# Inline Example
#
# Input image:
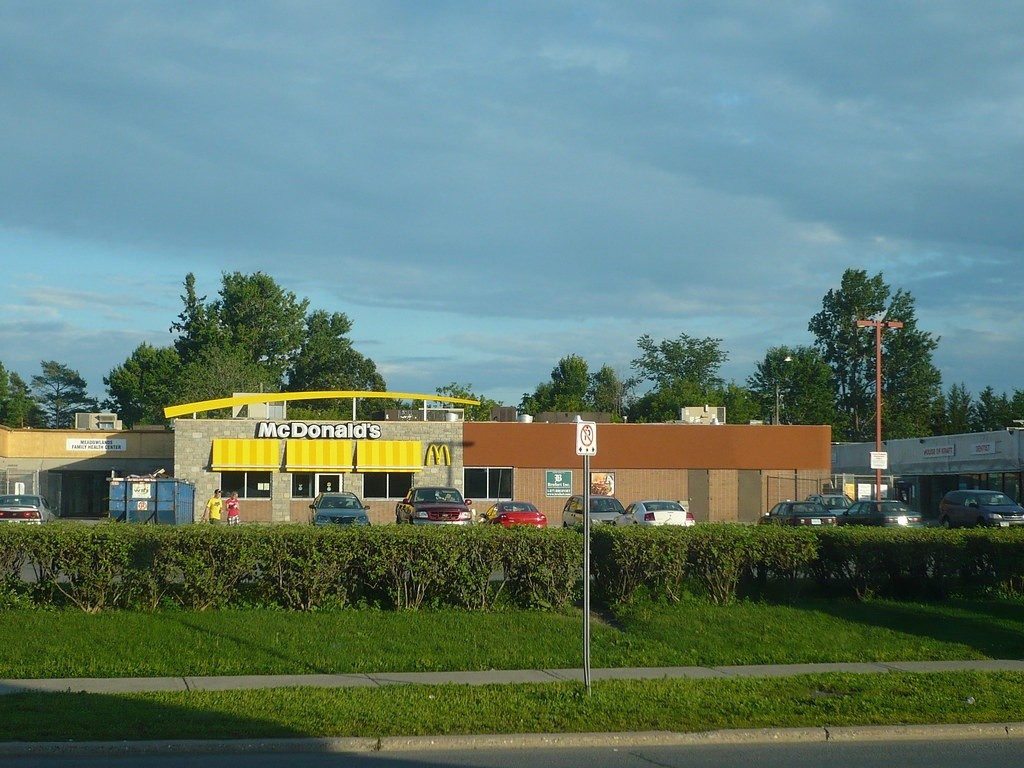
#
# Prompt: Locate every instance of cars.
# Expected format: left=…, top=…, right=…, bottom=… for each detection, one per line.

left=612, top=499, right=697, bottom=528
left=307, top=492, right=373, bottom=529
left=478, top=500, right=548, bottom=530
left=753, top=500, right=838, bottom=528
left=836, top=499, right=925, bottom=529
left=0, top=493, right=57, bottom=524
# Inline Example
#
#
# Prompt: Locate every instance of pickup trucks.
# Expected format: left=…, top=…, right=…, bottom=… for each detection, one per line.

left=393, top=486, right=475, bottom=525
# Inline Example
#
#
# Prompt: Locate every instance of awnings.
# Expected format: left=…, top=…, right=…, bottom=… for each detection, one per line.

left=356, top=440, right=424, bottom=472
left=285, top=439, right=354, bottom=472
left=211, top=439, right=281, bottom=471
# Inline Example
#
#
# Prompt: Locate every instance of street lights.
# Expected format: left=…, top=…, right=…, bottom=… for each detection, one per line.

left=855, top=320, right=904, bottom=502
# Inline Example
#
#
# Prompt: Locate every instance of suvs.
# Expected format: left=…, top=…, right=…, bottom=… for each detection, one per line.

left=802, top=494, right=868, bottom=525
left=937, top=489, right=1024, bottom=531
left=561, top=494, right=631, bottom=532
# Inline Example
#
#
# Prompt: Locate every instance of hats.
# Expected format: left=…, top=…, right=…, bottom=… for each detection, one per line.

left=215, top=489, right=222, bottom=494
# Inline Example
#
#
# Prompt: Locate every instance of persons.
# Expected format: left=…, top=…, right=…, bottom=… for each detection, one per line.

left=226, top=491, right=241, bottom=526
left=202, top=489, right=223, bottom=525
